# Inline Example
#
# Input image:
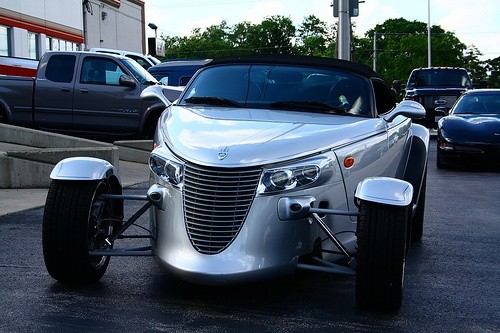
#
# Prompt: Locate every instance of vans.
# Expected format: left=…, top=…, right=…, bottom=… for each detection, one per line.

left=146, top=58, right=213, bottom=88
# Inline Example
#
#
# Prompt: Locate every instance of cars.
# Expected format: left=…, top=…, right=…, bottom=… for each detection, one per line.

left=435, top=87, right=500, bottom=170
left=41, top=53, right=428, bottom=313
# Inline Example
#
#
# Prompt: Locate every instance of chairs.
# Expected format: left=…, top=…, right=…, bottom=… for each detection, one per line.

left=52, top=57, right=67, bottom=82
left=95, top=61, right=106, bottom=83
left=269, top=69, right=304, bottom=102
left=224, top=71, right=262, bottom=100
left=302, top=74, right=338, bottom=103
left=327, top=77, right=397, bottom=117
left=467, top=101, right=487, bottom=113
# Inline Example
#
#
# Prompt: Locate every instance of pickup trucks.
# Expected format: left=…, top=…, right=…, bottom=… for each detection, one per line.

left=0, top=47, right=169, bottom=142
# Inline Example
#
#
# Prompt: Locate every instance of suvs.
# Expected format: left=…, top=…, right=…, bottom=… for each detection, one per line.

left=392, top=67, right=487, bottom=129
